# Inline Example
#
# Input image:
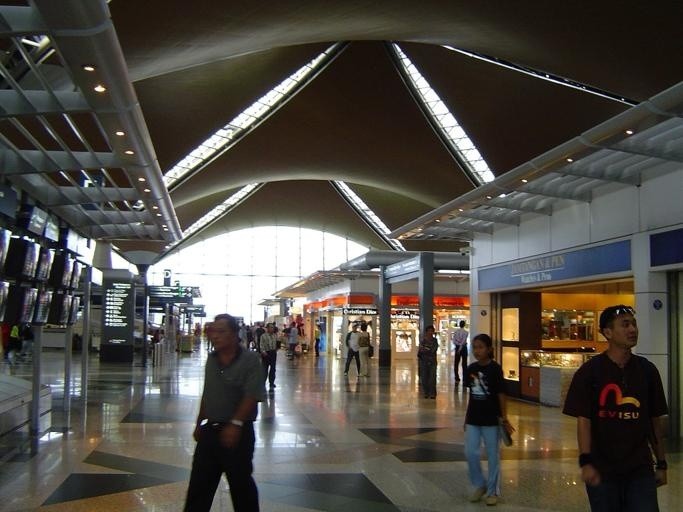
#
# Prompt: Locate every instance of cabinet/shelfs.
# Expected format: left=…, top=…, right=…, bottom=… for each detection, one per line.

left=542, top=308, right=608, bottom=343
left=490, top=295, right=542, bottom=405
left=519, top=348, right=601, bottom=407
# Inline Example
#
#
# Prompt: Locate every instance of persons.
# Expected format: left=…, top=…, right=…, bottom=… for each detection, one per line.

left=358, top=323, right=372, bottom=376
left=182, top=314, right=266, bottom=512
left=452, top=320, right=469, bottom=385
left=343, top=324, right=361, bottom=376
left=460, top=334, right=516, bottom=505
left=153, top=328, right=159, bottom=342
left=419, top=325, right=437, bottom=400
left=563, top=304, right=669, bottom=512
left=314, top=324, right=322, bottom=357
left=0, top=320, right=34, bottom=357
left=191, top=318, right=301, bottom=389
left=160, top=329, right=166, bottom=340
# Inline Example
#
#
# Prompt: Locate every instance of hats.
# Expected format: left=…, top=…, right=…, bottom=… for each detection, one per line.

left=352, top=323, right=358, bottom=328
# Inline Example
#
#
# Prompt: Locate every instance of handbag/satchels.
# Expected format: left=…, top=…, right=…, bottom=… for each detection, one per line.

left=294, top=345, right=302, bottom=355
left=499, top=416, right=513, bottom=446
left=360, top=335, right=369, bottom=347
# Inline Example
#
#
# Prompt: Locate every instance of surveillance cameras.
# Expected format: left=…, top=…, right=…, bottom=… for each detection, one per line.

left=459, top=247, right=472, bottom=257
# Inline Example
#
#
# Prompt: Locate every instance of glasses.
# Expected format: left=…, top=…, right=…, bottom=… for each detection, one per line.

left=610, top=306, right=634, bottom=318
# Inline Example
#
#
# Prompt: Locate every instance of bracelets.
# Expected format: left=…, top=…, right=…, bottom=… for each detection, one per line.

left=655, top=459, right=668, bottom=471
left=578, top=452, right=592, bottom=468
left=227, top=418, right=244, bottom=427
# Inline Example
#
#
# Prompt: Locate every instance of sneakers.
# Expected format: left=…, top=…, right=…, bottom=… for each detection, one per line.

left=425, top=394, right=436, bottom=399
left=270, top=383, right=276, bottom=387
left=344, top=372, right=370, bottom=377
left=469, top=488, right=486, bottom=502
left=485, top=494, right=498, bottom=505
